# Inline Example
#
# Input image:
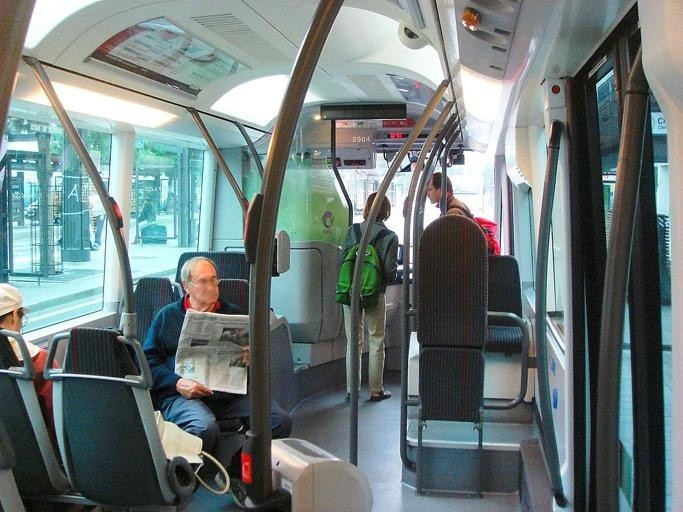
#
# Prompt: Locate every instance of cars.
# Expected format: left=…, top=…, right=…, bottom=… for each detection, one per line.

left=5, top=183, right=181, bottom=224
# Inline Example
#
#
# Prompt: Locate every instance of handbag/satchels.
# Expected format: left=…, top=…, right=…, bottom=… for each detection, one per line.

left=153, top=409, right=232, bottom=496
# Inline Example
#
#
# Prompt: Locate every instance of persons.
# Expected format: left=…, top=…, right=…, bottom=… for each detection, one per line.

left=425, top=170, right=473, bottom=223
left=138, top=257, right=291, bottom=493
left=89, top=190, right=107, bottom=251
left=130, top=197, right=157, bottom=245
left=336, top=191, right=398, bottom=402
left=0, top=284, right=63, bottom=448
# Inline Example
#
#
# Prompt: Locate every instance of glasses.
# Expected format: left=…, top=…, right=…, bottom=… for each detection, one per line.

left=187, top=279, right=219, bottom=288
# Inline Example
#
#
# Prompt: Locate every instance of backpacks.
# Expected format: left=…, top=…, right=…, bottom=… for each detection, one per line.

left=334, top=221, right=394, bottom=310
left=447, top=202, right=501, bottom=258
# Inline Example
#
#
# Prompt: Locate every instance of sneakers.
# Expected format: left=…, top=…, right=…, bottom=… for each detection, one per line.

left=346, top=392, right=359, bottom=403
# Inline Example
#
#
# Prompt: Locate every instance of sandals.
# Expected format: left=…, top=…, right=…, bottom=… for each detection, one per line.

left=370, top=390, right=391, bottom=401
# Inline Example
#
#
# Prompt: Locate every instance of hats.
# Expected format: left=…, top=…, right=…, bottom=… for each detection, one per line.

left=0, top=283, right=31, bottom=318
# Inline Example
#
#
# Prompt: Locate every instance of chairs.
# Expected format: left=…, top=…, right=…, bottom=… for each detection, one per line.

left=487, top=255, right=530, bottom=409
